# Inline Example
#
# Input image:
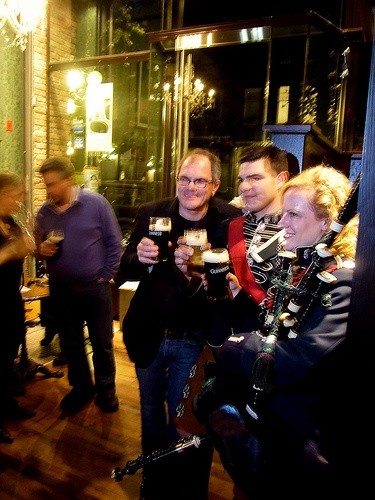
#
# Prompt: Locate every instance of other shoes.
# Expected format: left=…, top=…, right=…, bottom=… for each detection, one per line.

left=41, top=336, right=53, bottom=346
left=0, top=432, right=12, bottom=443
left=3, top=405, right=36, bottom=418
left=60, top=382, right=95, bottom=413
left=95, top=386, right=119, bottom=413
left=53, top=357, right=68, bottom=366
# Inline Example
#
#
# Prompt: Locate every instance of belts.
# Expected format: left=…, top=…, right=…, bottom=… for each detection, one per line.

left=163, top=325, right=209, bottom=343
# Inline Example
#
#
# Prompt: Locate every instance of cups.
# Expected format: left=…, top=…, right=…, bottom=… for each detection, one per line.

left=50, top=230, right=65, bottom=256
left=184, top=229, right=208, bottom=278
left=148, top=216, right=172, bottom=262
left=201, top=249, right=230, bottom=301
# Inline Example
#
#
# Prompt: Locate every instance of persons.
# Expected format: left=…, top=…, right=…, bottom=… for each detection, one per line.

left=175, top=143, right=300, bottom=379
left=117, top=147, right=245, bottom=499
left=201, top=164, right=362, bottom=500
left=33, top=157, right=123, bottom=415
left=0, top=170, right=38, bottom=445
left=39, top=299, right=67, bottom=367
left=226, top=151, right=300, bottom=213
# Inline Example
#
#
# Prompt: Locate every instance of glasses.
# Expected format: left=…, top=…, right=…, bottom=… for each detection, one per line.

left=176, top=176, right=216, bottom=188
